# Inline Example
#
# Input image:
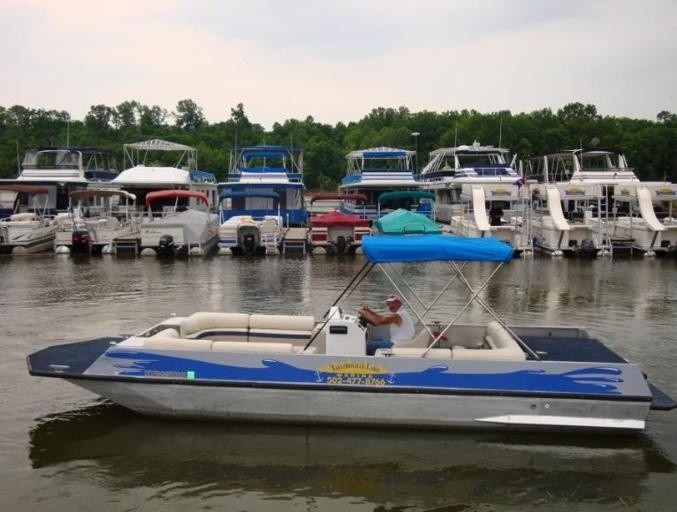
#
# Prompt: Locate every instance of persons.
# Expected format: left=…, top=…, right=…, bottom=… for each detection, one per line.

left=489, top=201, right=504, bottom=225
left=358, top=294, right=415, bottom=354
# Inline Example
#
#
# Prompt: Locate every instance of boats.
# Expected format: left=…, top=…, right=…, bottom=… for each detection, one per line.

left=24, top=207, right=676, bottom=436
left=28, top=415, right=675, bottom=503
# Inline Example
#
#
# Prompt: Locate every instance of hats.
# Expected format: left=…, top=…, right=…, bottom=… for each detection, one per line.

left=384, top=294, right=399, bottom=302
